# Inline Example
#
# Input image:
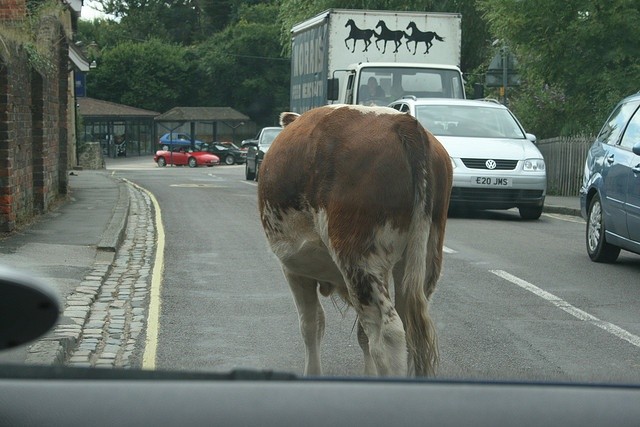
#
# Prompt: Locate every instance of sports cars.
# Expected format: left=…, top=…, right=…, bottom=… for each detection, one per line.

left=153, top=144, right=219, bottom=167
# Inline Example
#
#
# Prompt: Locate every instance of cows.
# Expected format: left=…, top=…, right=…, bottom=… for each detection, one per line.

left=255, top=102, right=454, bottom=379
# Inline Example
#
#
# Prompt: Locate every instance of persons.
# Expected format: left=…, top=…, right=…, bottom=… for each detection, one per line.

left=357, top=76, right=387, bottom=107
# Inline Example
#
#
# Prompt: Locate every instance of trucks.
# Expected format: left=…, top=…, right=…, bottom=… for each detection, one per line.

left=289, top=3, right=485, bottom=113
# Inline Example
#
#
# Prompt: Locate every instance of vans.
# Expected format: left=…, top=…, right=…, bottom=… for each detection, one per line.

left=384, top=95, right=547, bottom=218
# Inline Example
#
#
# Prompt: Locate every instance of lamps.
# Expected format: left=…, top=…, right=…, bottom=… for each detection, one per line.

left=52, top=31, right=83, bottom=48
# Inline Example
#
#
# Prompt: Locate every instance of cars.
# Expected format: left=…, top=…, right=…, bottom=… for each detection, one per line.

left=158, top=131, right=210, bottom=152
left=245, top=125, right=284, bottom=182
left=196, top=143, right=246, bottom=165
left=576, top=88, right=640, bottom=265
